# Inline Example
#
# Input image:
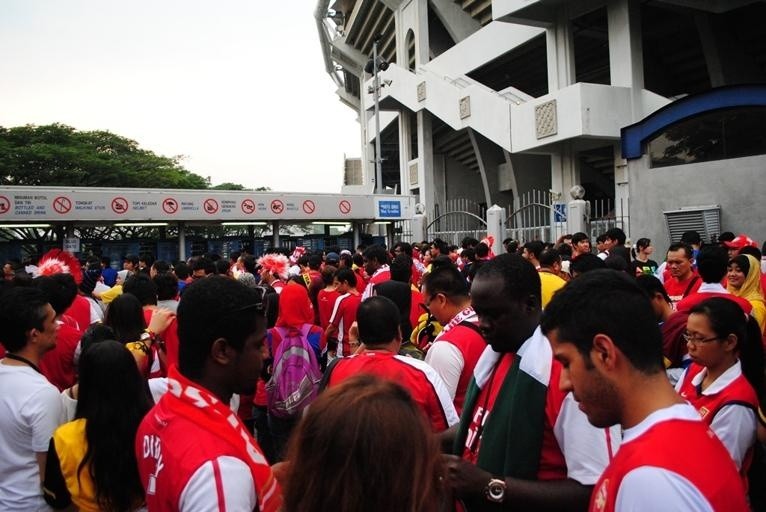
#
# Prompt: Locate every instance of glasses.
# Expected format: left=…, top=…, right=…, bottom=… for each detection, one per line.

left=240, top=285, right=269, bottom=318
left=682, top=332, right=720, bottom=345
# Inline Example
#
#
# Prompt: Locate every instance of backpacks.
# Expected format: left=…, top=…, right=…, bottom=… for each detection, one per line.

left=267, top=324, right=323, bottom=420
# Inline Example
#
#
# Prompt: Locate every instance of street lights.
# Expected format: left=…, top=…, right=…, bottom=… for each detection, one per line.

left=364, top=31, right=389, bottom=192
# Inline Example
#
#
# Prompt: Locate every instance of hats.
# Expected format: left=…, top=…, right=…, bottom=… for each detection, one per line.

left=122, top=254, right=139, bottom=263
left=238, top=272, right=258, bottom=290
left=36, top=249, right=85, bottom=285
left=257, top=254, right=291, bottom=281
left=80, top=269, right=99, bottom=295
left=309, top=255, right=323, bottom=264
left=724, top=234, right=758, bottom=248
left=326, top=253, right=343, bottom=262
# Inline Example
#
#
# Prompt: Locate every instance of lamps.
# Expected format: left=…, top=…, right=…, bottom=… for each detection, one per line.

left=568, top=185, right=586, bottom=199
left=413, top=203, right=426, bottom=215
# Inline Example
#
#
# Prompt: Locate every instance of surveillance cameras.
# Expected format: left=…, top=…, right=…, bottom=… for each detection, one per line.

left=375, top=54, right=390, bottom=71
left=363, top=60, right=373, bottom=73
